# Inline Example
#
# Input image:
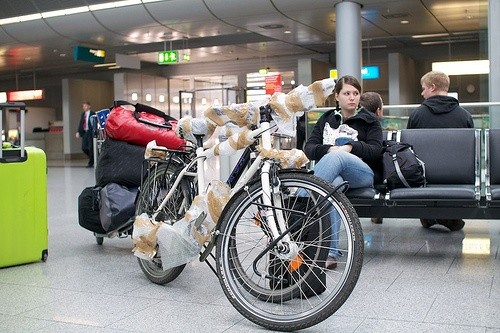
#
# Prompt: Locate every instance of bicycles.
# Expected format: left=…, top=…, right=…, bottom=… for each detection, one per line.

left=131, top=79, right=366, bottom=332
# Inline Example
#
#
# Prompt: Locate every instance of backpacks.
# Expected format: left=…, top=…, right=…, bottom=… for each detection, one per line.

left=381, top=140, right=425, bottom=191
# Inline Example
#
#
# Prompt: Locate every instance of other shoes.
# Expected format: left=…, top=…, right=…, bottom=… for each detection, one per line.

left=438, top=219, right=465, bottom=230
left=87, top=164, right=94, bottom=168
left=371, top=218, right=383, bottom=224
left=419, top=218, right=436, bottom=227
left=325, top=255, right=337, bottom=269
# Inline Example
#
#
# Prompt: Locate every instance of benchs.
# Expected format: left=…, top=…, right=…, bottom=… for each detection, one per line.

left=297, top=127, right=500, bottom=220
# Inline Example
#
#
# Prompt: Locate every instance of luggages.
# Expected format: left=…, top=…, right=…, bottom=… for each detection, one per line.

left=0, top=102, right=49, bottom=268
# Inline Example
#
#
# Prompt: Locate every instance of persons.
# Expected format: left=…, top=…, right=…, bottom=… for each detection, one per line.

left=406, top=72, right=474, bottom=232
left=288, top=75, right=386, bottom=271
left=75, top=102, right=97, bottom=168
left=360, top=91, right=384, bottom=120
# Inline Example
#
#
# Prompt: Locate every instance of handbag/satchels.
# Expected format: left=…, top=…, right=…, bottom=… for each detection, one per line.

left=99, top=182, right=141, bottom=235
left=269, top=196, right=330, bottom=298
left=78, top=187, right=105, bottom=235
left=95, top=138, right=149, bottom=187
left=105, top=101, right=186, bottom=150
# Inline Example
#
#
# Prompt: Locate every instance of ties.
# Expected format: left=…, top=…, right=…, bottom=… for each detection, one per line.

left=83, top=114, right=87, bottom=128
